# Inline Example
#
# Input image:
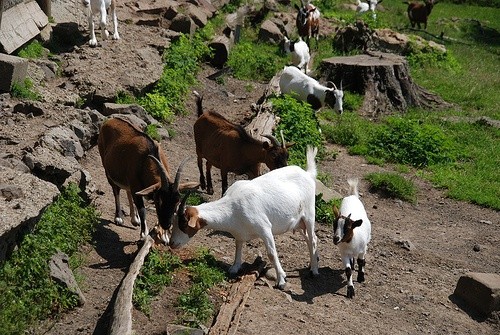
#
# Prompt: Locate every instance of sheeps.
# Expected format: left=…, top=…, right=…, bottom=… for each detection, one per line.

left=400, top=0, right=440, bottom=31
left=331, top=175, right=373, bottom=299
left=278, top=64, right=346, bottom=136
left=278, top=36, right=311, bottom=76
left=93, top=116, right=201, bottom=246
left=168, top=143, right=322, bottom=291
left=292, top=0, right=321, bottom=55
left=79, top=0, right=124, bottom=48
left=190, top=89, right=298, bottom=199
left=352, top=0, right=384, bottom=20
